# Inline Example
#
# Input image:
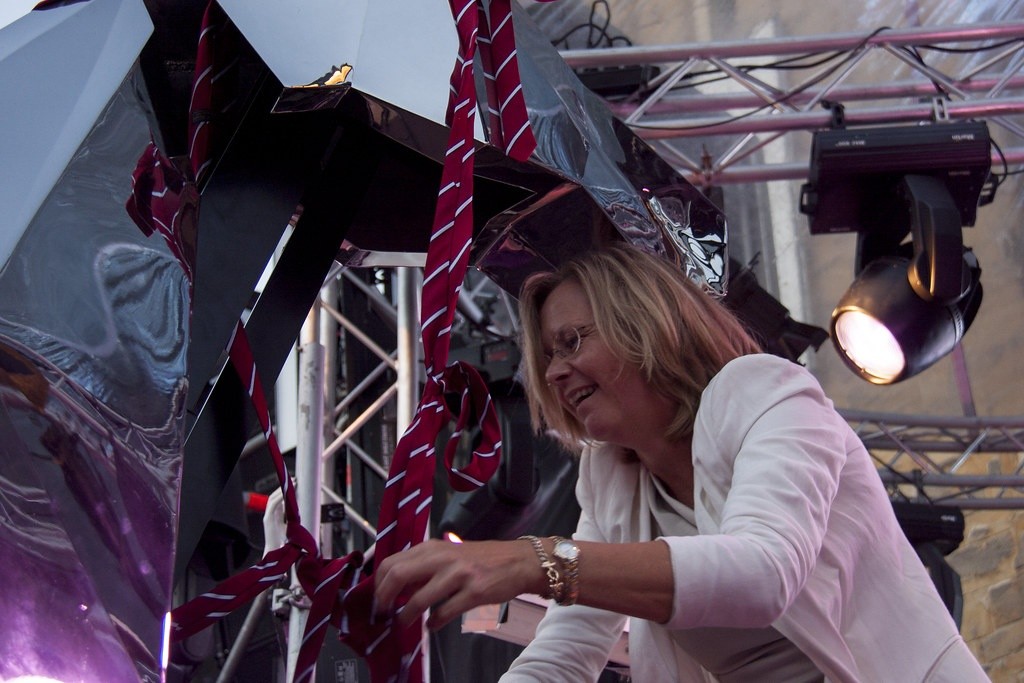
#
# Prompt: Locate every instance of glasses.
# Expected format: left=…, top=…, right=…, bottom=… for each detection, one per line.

left=544, top=322, right=599, bottom=371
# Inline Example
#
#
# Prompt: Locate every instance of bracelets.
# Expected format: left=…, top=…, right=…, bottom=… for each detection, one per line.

left=517, top=534, right=563, bottom=600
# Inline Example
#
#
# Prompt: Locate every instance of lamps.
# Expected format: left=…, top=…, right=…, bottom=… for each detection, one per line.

left=824, top=170, right=985, bottom=386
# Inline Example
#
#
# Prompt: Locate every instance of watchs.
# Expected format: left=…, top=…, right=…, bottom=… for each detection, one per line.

left=549, top=536, right=580, bottom=606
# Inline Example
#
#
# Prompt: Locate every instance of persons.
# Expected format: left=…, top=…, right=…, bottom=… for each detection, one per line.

left=373, top=242, right=993, bottom=683
